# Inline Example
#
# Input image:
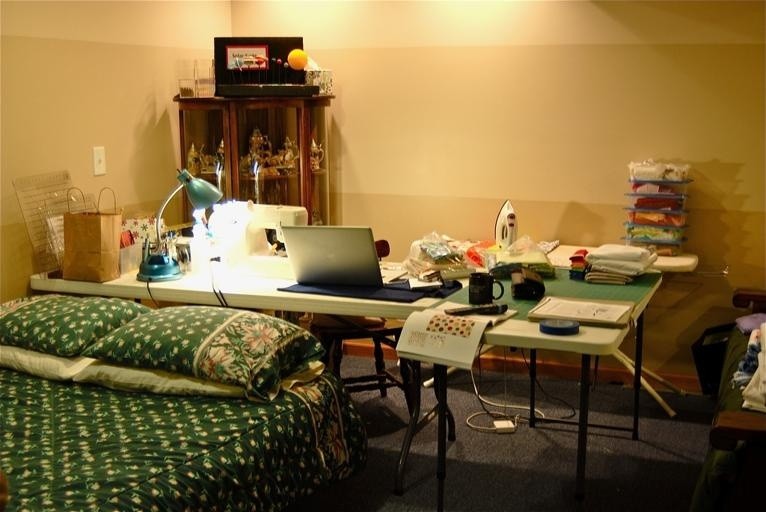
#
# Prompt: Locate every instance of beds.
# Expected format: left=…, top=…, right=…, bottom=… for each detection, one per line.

left=1, top=365, right=369, bottom=512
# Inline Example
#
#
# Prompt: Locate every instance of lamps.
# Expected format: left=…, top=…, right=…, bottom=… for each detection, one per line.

left=136, top=168, right=223, bottom=282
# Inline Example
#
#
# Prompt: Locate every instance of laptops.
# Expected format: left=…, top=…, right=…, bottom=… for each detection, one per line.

left=283, top=226, right=406, bottom=291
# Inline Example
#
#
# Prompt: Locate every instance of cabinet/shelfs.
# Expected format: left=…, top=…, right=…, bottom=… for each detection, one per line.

left=173, top=94, right=337, bottom=226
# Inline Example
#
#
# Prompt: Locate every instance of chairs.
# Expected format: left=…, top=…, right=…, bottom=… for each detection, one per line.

left=284, top=312, right=414, bottom=416
left=685, top=287, right=766, bottom=509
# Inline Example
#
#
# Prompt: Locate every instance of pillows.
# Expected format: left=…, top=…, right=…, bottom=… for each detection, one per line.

left=83, top=306, right=325, bottom=397
left=0, top=293, right=153, bottom=358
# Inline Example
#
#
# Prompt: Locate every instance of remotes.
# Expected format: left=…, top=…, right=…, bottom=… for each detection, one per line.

left=444, top=303, right=510, bottom=319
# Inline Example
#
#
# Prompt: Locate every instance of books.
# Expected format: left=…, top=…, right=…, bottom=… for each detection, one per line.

left=529, top=294, right=634, bottom=327
left=396, top=300, right=518, bottom=370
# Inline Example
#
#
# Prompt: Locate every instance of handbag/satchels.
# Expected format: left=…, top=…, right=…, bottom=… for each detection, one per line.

left=511, top=267, right=545, bottom=301
left=62, top=188, right=123, bottom=282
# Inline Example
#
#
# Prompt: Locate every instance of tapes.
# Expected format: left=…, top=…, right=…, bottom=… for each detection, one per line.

left=539, top=320, right=579, bottom=335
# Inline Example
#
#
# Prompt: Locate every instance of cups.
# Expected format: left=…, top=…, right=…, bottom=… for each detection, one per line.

left=312, top=71, right=333, bottom=94
left=179, top=80, right=194, bottom=98
left=192, top=59, right=215, bottom=98
left=469, top=273, right=504, bottom=304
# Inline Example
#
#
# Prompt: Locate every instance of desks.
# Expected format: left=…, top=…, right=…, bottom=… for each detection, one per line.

left=30, top=242, right=490, bottom=497
left=424, top=272, right=663, bottom=512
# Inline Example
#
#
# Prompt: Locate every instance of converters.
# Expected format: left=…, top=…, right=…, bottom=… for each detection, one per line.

left=494, top=420, right=515, bottom=434
